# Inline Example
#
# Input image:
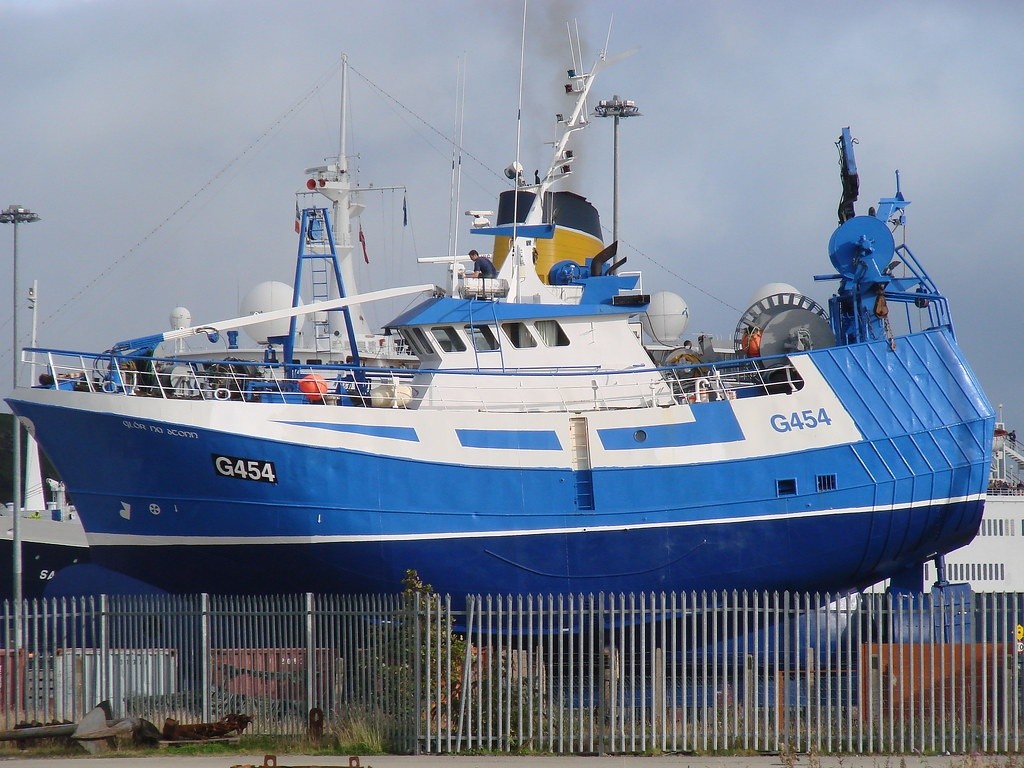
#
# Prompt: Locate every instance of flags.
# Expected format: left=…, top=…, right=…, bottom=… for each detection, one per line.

left=295, top=200, right=300, bottom=234
left=403, top=195, right=407, bottom=227
left=359, top=225, right=369, bottom=264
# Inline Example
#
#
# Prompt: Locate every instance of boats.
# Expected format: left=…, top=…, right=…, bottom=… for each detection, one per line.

left=4, top=0, right=996, bottom=640
left=687, top=402, right=1024, bottom=665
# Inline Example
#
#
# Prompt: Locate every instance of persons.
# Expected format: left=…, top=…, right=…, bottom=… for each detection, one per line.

left=465, top=250, right=496, bottom=301
left=1009, top=430, right=1016, bottom=450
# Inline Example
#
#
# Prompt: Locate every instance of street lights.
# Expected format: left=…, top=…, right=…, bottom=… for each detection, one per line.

left=0, top=202, right=40, bottom=649
